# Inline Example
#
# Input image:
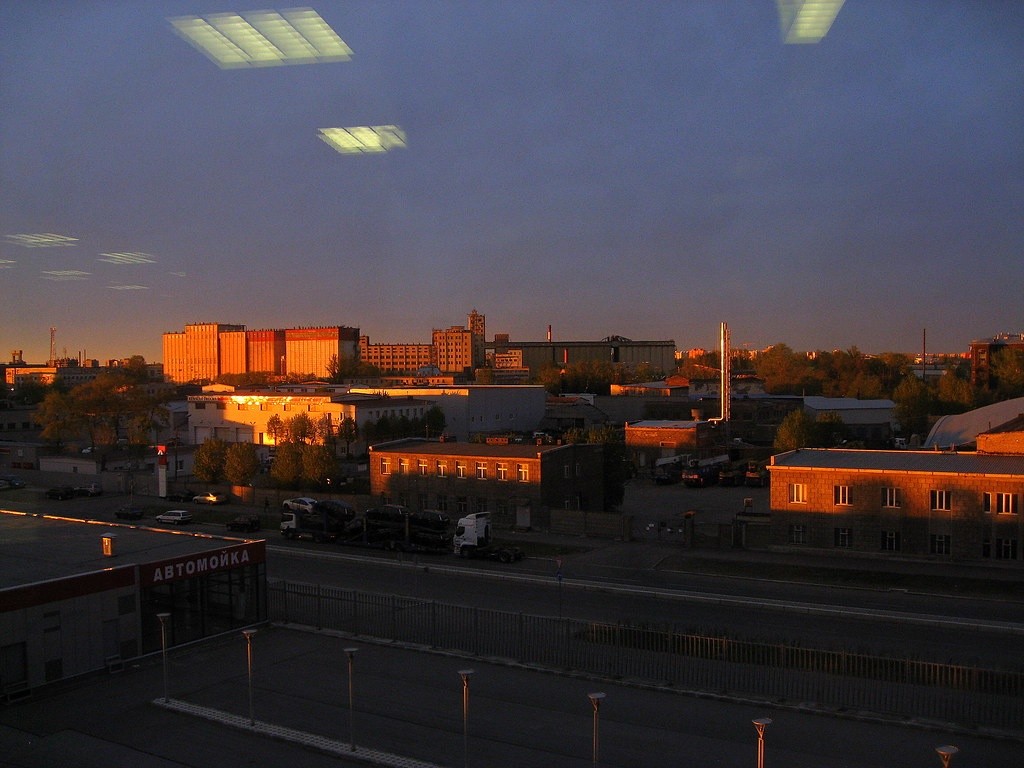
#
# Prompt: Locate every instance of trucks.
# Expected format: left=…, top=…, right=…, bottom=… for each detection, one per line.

left=656, top=449, right=770, bottom=488
left=450, top=512, right=528, bottom=564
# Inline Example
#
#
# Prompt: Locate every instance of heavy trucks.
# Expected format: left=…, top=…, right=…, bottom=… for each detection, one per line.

left=278, top=496, right=456, bottom=556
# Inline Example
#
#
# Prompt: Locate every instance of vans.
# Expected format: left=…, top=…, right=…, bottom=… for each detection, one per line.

left=1, top=474, right=26, bottom=489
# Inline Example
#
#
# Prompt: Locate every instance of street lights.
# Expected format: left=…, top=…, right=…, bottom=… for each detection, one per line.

left=587, top=692, right=608, bottom=768
left=750, top=717, right=773, bottom=768
left=456, top=669, right=480, bottom=768
left=156, top=610, right=171, bottom=705
left=241, top=628, right=258, bottom=725
left=343, top=646, right=359, bottom=754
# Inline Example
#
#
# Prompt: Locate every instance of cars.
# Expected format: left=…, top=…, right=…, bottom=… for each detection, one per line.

left=114, top=506, right=145, bottom=519
left=156, top=510, right=193, bottom=525
left=44, top=481, right=101, bottom=501
left=226, top=515, right=261, bottom=533
left=364, top=504, right=411, bottom=524
left=313, top=499, right=357, bottom=522
left=281, top=498, right=316, bottom=514
left=408, top=509, right=451, bottom=531
left=192, top=492, right=228, bottom=505
